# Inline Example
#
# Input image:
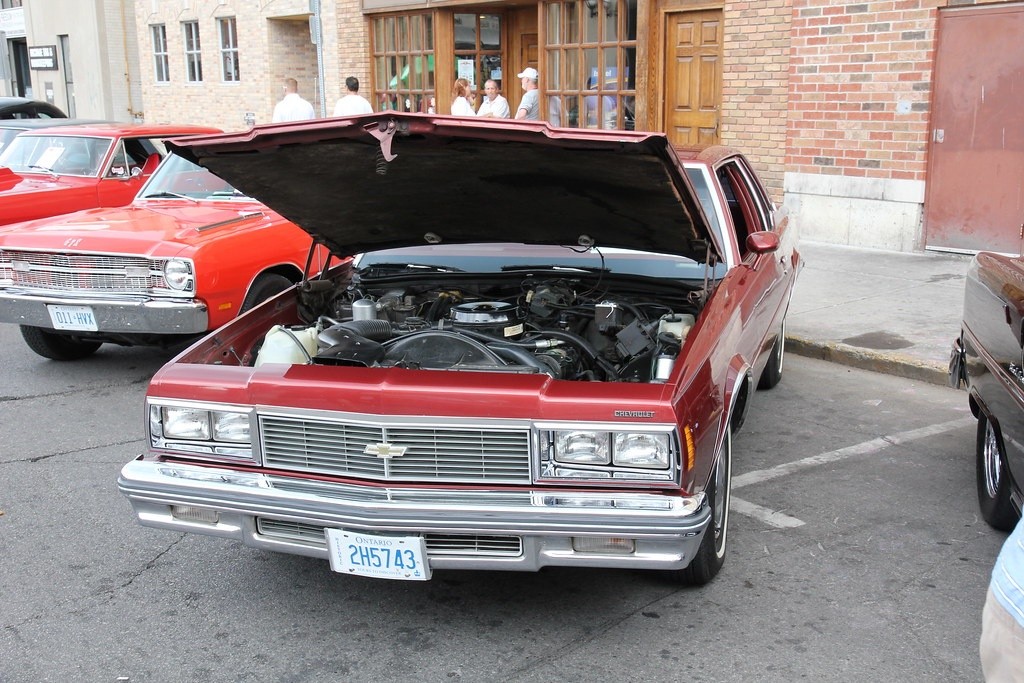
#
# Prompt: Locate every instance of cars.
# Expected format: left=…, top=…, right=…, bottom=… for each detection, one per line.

left=948, top=255, right=1024, bottom=531
left=0, top=96, right=226, bottom=234
left=115, top=109, right=805, bottom=589
left=0, top=144, right=352, bottom=364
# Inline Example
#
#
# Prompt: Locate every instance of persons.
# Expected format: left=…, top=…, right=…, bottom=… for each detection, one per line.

left=476, top=79, right=511, bottom=119
left=578, top=77, right=614, bottom=131
left=271, top=78, right=316, bottom=124
left=515, top=68, right=541, bottom=120
left=333, top=77, right=374, bottom=118
left=548, top=94, right=569, bottom=128
left=969, top=505, right=1024, bottom=683
left=450, top=78, right=478, bottom=117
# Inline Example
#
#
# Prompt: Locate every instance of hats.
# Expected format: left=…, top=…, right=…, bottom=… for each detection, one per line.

left=517, top=68, right=538, bottom=79
